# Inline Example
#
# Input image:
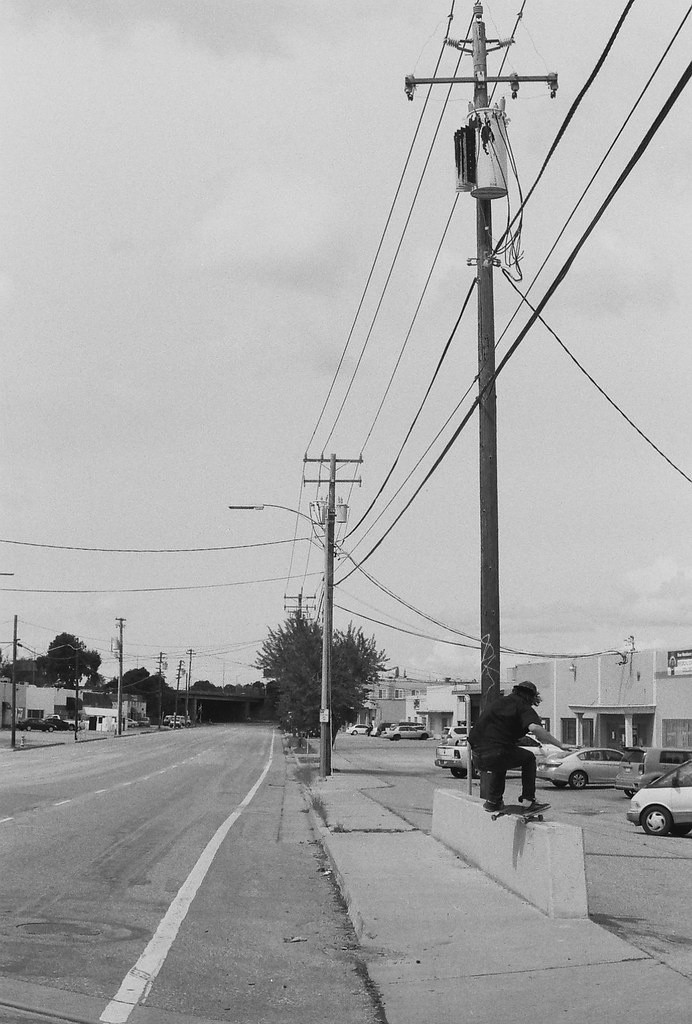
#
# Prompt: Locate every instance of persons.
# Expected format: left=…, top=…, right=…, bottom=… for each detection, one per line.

left=366, top=723, right=373, bottom=736
left=468, top=681, right=583, bottom=811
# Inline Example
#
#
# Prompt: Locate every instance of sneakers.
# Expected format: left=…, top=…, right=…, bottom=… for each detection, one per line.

left=523, top=803, right=551, bottom=816
left=483, top=799, right=505, bottom=810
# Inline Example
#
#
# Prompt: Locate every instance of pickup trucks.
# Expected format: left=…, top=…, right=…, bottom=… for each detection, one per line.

left=435, top=737, right=489, bottom=779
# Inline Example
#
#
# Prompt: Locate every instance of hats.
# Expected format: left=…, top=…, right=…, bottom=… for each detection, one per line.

left=514, top=682, right=536, bottom=697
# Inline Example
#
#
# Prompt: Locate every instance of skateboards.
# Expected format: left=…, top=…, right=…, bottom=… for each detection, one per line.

left=485, top=803, right=551, bottom=824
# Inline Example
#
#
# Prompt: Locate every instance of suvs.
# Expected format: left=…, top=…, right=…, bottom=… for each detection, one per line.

left=164, top=715, right=191, bottom=728
left=441, top=726, right=473, bottom=744
left=139, top=717, right=151, bottom=727
left=170, top=716, right=181, bottom=728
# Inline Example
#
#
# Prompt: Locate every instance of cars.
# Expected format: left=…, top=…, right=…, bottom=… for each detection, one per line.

left=16, top=718, right=57, bottom=732
left=128, top=718, right=140, bottom=727
left=39, top=718, right=75, bottom=732
left=346, top=724, right=370, bottom=735
left=380, top=726, right=430, bottom=741
left=536, top=747, right=627, bottom=789
left=507, top=734, right=548, bottom=771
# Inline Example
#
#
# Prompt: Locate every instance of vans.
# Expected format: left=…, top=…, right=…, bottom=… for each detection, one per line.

left=615, top=747, right=692, bottom=798
left=627, top=759, right=692, bottom=836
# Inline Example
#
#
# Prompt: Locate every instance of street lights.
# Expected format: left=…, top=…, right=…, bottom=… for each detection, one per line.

left=227, top=504, right=328, bottom=779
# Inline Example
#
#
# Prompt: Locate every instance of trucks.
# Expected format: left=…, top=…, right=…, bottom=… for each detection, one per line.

left=42, top=715, right=79, bottom=731
